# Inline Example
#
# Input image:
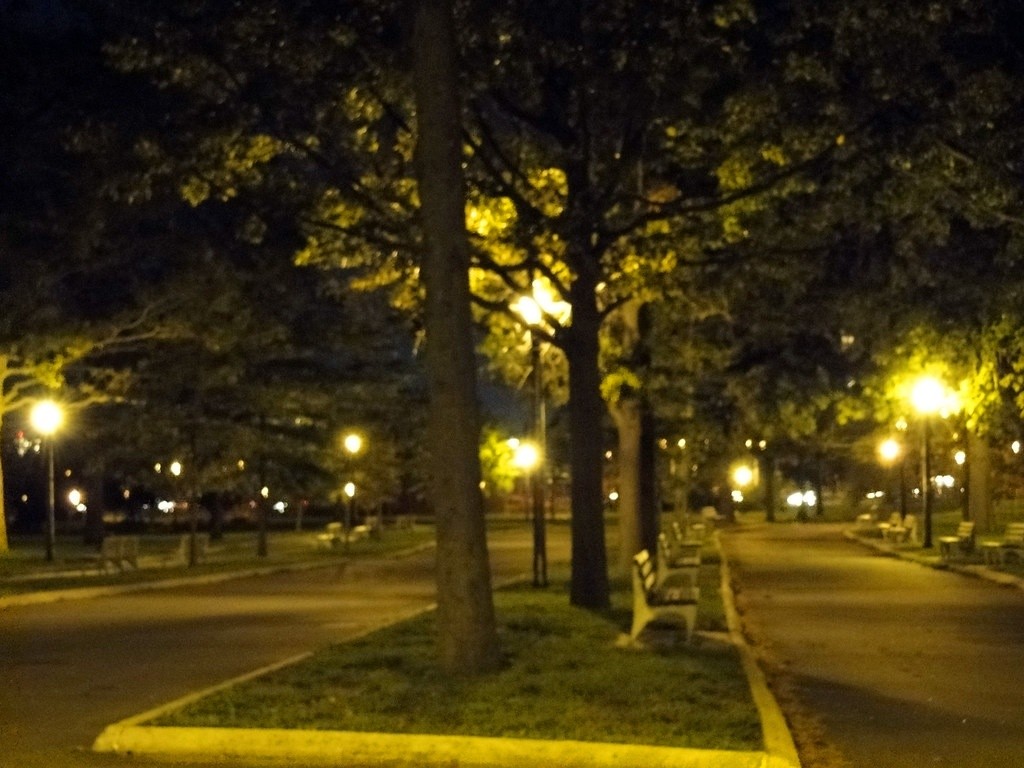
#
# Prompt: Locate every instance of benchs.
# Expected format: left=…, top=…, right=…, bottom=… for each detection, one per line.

left=74, top=536, right=140, bottom=578
left=317, top=512, right=418, bottom=548
left=671, top=522, right=703, bottom=557
left=653, top=533, right=699, bottom=592
left=856, top=505, right=878, bottom=527
left=980, top=523, right=1024, bottom=566
left=890, top=513, right=918, bottom=544
left=686, top=506, right=721, bottom=536
left=938, top=521, right=975, bottom=561
left=631, top=549, right=700, bottom=639
left=170, top=534, right=210, bottom=565
left=878, top=512, right=902, bottom=540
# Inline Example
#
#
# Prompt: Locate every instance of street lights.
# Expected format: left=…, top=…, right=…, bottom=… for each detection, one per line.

left=334, top=426, right=371, bottom=531
left=912, top=374, right=950, bottom=548
left=512, top=284, right=569, bottom=592
left=25, top=394, right=70, bottom=566
